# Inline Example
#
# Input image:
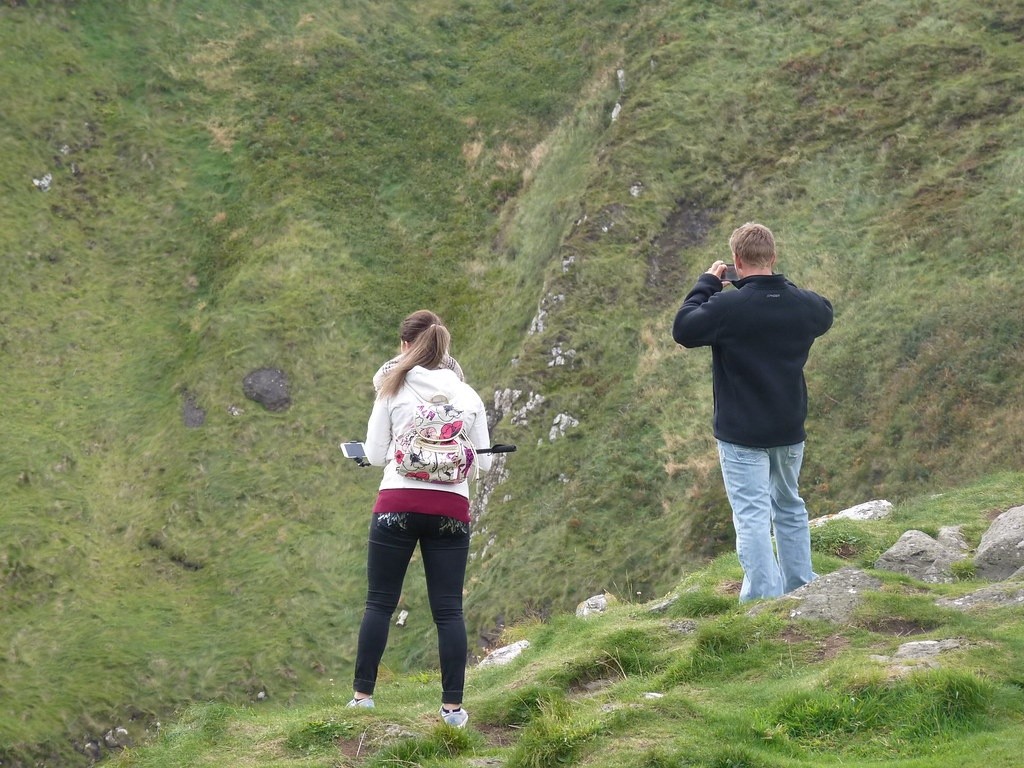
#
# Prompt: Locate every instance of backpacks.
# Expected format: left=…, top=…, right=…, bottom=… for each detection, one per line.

left=391, top=378, right=480, bottom=485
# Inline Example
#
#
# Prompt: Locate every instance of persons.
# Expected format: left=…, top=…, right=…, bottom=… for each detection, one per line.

left=345, top=310, right=492, bottom=731
left=672, top=223, right=834, bottom=606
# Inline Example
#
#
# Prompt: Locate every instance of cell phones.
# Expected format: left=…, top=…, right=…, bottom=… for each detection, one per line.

left=340, top=442, right=366, bottom=459
left=720, top=264, right=738, bottom=280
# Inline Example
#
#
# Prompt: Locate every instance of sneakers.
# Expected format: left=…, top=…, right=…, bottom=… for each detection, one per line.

left=346, top=697, right=374, bottom=708
left=438, top=704, right=468, bottom=730
